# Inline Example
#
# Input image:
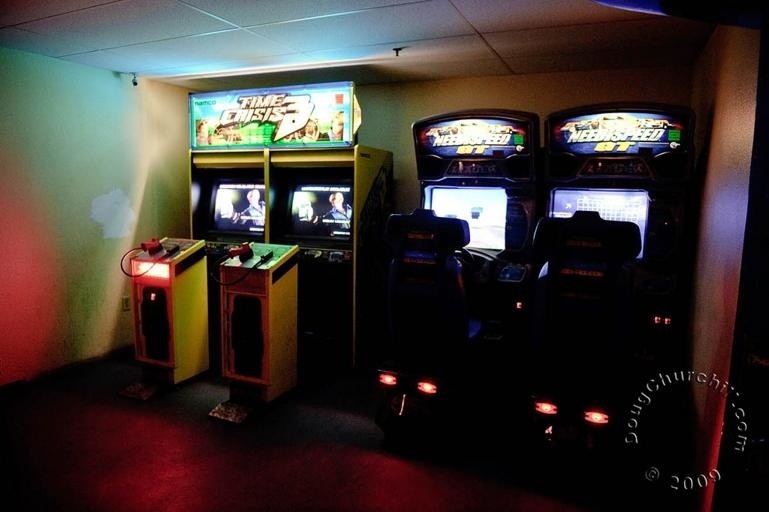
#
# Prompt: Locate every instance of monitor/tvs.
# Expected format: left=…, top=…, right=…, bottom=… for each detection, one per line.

left=209, top=181, right=266, bottom=233
left=287, top=184, right=353, bottom=244
left=550, top=189, right=648, bottom=258
left=430, top=187, right=508, bottom=250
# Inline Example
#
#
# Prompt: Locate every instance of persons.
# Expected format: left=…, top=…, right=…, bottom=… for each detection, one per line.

left=298, top=191, right=353, bottom=226
left=221, top=188, right=266, bottom=228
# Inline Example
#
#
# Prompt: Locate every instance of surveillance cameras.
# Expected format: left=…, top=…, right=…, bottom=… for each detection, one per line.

left=132, top=74, right=138, bottom=87
